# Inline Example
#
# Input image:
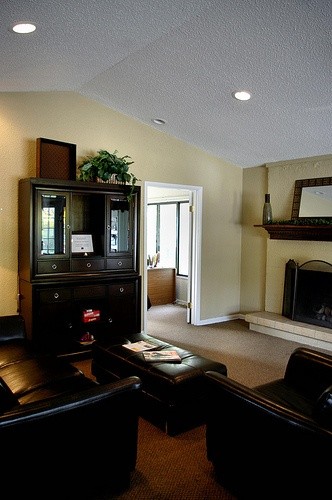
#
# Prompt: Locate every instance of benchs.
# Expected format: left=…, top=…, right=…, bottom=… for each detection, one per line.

left=90, top=333, right=227, bottom=436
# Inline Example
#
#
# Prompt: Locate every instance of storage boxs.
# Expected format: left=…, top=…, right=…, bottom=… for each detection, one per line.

left=34, top=138, right=77, bottom=180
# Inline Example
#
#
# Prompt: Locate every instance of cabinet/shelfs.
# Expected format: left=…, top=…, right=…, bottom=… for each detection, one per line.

left=18, top=177, right=141, bottom=363
left=147, top=267, right=176, bottom=305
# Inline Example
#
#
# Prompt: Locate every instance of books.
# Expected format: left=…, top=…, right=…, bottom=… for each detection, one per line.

left=142, top=350, right=181, bottom=362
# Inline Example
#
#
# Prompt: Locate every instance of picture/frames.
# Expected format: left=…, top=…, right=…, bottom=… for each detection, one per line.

left=291, top=177, right=332, bottom=219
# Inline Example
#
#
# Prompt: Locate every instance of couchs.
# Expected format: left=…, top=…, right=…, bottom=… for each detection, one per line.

left=203, top=346, right=332, bottom=500
left=0, top=314, right=142, bottom=500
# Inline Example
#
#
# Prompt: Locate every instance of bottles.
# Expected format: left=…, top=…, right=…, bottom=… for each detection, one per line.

left=263, top=193, right=273, bottom=224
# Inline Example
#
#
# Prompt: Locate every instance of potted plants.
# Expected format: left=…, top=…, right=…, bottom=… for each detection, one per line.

left=77, top=149, right=141, bottom=203
